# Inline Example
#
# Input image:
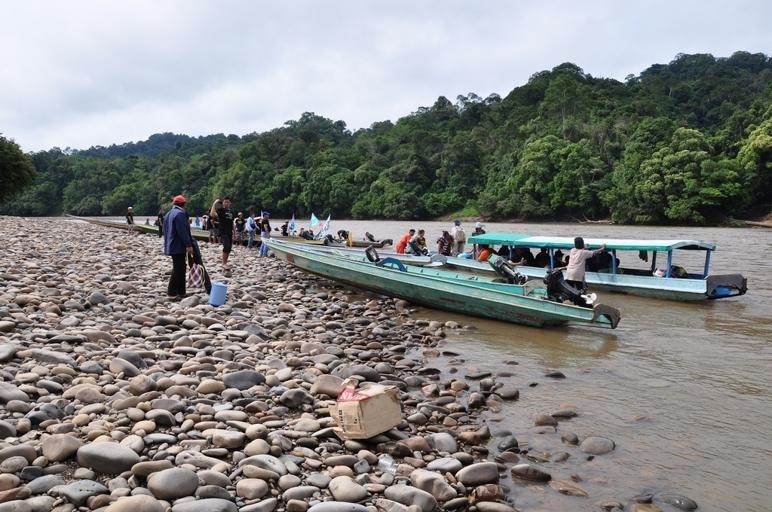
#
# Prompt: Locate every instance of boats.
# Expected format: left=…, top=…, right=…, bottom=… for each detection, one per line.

left=259, top=237, right=624, bottom=329
left=436, top=229, right=747, bottom=302
left=65, top=213, right=449, bottom=297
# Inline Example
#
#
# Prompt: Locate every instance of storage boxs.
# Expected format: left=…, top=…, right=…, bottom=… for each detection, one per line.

left=329, top=380, right=403, bottom=441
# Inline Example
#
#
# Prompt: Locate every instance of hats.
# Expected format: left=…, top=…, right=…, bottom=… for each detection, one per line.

left=173, top=195, right=186, bottom=203
left=263, top=211, right=270, bottom=215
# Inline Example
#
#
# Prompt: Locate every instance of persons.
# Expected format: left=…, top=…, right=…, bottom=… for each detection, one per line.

left=396, top=220, right=607, bottom=309
left=125, top=197, right=349, bottom=302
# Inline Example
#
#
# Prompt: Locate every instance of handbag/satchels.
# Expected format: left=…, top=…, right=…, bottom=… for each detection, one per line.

left=186, top=250, right=204, bottom=288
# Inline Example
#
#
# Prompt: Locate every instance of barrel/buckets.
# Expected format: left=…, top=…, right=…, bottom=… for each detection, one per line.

left=208, top=282, right=228, bottom=305
